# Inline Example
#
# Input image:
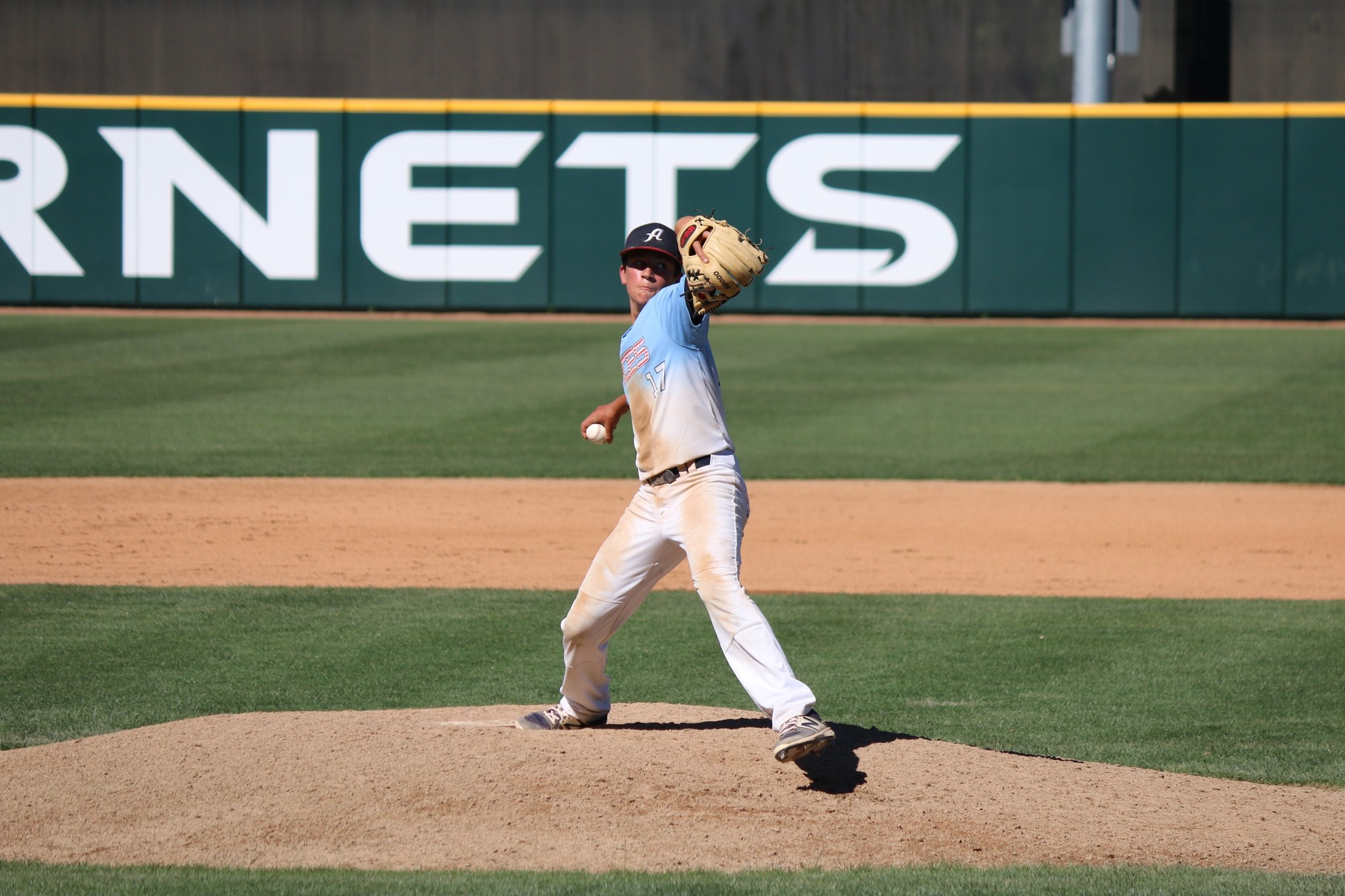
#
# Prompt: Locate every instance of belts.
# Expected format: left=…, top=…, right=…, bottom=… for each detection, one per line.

left=647, top=455, right=710, bottom=484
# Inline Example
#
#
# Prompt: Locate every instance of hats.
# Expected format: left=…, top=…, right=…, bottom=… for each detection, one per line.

left=620, top=223, right=682, bottom=264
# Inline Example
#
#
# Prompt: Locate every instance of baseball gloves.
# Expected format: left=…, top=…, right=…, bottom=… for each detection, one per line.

left=675, top=206, right=775, bottom=319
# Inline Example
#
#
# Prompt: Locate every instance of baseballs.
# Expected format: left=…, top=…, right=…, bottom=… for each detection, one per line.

left=586, top=423, right=607, bottom=445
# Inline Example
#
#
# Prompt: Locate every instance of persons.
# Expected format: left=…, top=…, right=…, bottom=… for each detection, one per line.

left=511, top=215, right=835, bottom=763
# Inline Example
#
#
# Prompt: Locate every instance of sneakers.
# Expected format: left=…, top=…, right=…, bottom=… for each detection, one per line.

left=773, top=709, right=835, bottom=763
left=514, top=704, right=607, bottom=730
